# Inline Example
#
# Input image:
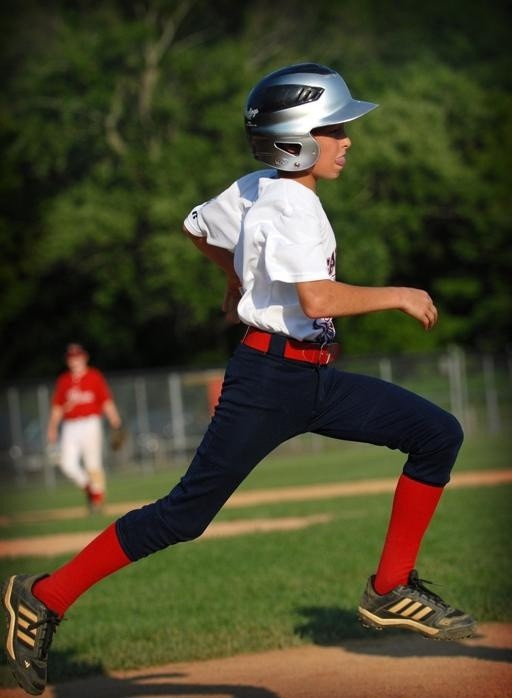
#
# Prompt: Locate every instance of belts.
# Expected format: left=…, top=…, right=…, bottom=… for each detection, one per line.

left=242, top=326, right=342, bottom=366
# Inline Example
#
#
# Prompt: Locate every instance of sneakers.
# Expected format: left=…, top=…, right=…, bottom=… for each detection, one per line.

left=356, top=569, right=479, bottom=641
left=1, top=572, right=65, bottom=696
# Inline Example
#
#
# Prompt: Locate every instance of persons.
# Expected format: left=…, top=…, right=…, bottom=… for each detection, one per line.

left=45, top=339, right=122, bottom=513
left=0, top=60, right=482, bottom=695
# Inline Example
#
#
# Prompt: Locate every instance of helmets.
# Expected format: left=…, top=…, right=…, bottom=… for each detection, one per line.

left=244, top=63, right=379, bottom=172
